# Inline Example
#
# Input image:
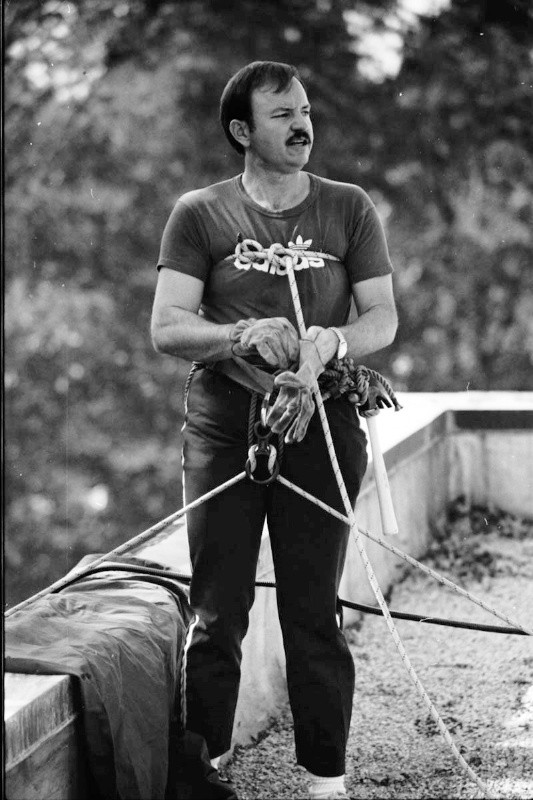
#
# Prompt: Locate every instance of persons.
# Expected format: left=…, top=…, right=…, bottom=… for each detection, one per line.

left=150, top=61, right=398, bottom=800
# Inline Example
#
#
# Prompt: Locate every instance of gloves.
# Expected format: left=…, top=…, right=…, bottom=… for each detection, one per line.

left=230, top=318, right=300, bottom=371
left=267, top=338, right=326, bottom=445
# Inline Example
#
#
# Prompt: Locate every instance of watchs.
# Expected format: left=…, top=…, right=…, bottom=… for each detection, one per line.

left=331, top=327, right=348, bottom=360
